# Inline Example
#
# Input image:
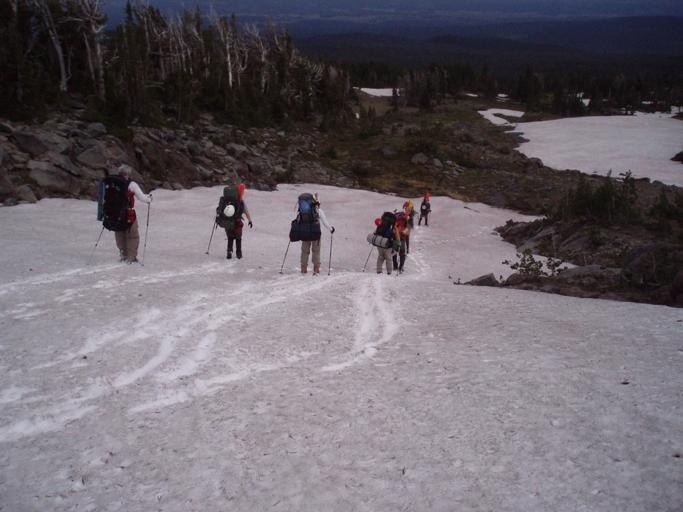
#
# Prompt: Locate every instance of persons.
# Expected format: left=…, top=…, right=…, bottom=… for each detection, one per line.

left=116, top=164, right=153, bottom=264
left=299, top=193, right=335, bottom=274
left=375, top=192, right=433, bottom=275
left=225, top=181, right=253, bottom=259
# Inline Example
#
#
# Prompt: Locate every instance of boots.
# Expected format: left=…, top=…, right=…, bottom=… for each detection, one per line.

left=391, top=252, right=398, bottom=271
left=418, top=218, right=428, bottom=226
left=398, top=255, right=405, bottom=273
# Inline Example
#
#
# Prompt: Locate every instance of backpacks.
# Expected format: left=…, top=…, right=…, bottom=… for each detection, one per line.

left=95, top=174, right=134, bottom=233
left=213, top=185, right=244, bottom=231
left=372, top=211, right=397, bottom=239
left=287, top=193, right=321, bottom=242
